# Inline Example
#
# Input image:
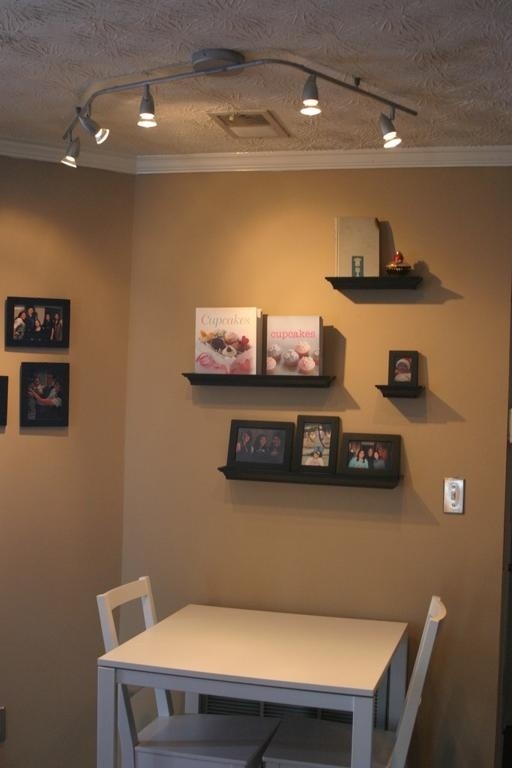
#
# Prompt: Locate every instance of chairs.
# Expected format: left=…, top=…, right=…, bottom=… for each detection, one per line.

left=96, top=575, right=283, bottom=768
left=262, top=595, right=448, bottom=768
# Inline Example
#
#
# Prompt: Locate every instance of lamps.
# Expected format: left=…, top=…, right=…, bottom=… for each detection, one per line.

left=60, top=48, right=418, bottom=168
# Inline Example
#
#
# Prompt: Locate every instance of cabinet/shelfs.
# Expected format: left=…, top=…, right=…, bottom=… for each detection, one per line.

left=181, top=276, right=427, bottom=489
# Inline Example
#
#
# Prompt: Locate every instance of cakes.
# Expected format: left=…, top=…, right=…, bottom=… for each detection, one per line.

left=266, top=342, right=320, bottom=375
left=197, top=328, right=256, bottom=376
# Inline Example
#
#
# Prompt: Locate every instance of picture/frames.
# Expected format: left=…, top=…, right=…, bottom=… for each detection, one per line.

left=0, top=375, right=8, bottom=426
left=5, top=294, right=70, bottom=349
left=19, top=361, right=69, bottom=427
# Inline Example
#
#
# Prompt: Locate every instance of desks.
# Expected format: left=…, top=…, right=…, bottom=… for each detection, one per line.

left=96, top=603, right=410, bottom=768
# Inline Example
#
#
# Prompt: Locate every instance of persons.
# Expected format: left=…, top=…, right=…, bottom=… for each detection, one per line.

left=32, top=378, right=67, bottom=407
left=12, top=304, right=63, bottom=343
left=394, top=356, right=412, bottom=383
left=235, top=429, right=393, bottom=473
left=31, top=373, right=46, bottom=397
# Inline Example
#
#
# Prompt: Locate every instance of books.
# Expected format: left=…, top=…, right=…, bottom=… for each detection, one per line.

left=332, top=213, right=382, bottom=276
left=266, top=314, right=326, bottom=376
left=194, top=305, right=264, bottom=374
